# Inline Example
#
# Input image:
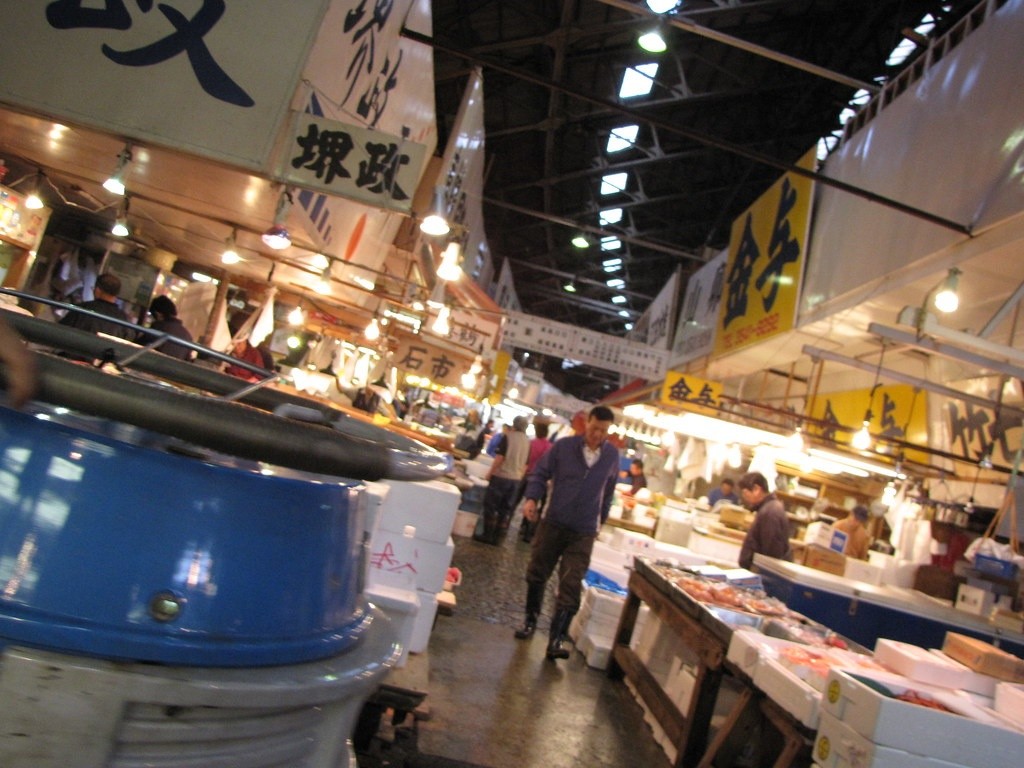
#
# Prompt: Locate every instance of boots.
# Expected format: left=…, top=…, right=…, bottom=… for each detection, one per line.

left=546, top=609, right=577, bottom=658
left=515, top=591, right=542, bottom=640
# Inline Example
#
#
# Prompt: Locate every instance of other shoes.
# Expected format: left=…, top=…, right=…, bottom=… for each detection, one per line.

left=473, top=533, right=505, bottom=547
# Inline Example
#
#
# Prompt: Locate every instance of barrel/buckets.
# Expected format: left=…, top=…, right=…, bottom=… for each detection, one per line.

left=454, top=510, right=478, bottom=539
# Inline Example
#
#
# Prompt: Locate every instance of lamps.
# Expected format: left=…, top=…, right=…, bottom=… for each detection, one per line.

left=103, top=144, right=137, bottom=195
left=934, top=266, right=962, bottom=314
left=426, top=279, right=448, bottom=308
left=638, top=25, right=667, bottom=52
left=421, top=188, right=449, bottom=236
left=264, top=188, right=291, bottom=249
left=572, top=232, right=592, bottom=249
left=111, top=198, right=131, bottom=237
left=435, top=229, right=464, bottom=282
left=564, top=279, right=575, bottom=292
left=220, top=236, right=239, bottom=264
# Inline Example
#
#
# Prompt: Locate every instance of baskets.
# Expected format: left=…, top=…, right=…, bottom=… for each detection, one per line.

left=974, top=554, right=1019, bottom=579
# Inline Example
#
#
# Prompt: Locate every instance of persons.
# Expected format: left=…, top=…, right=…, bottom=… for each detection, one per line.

left=737, top=472, right=794, bottom=575
left=56, top=273, right=137, bottom=367
left=393, top=387, right=512, bottom=459
left=135, top=294, right=194, bottom=363
left=831, top=505, right=871, bottom=562
left=709, top=479, right=740, bottom=508
left=513, top=405, right=620, bottom=662
left=473, top=415, right=531, bottom=549
left=224, top=337, right=265, bottom=383
left=618, top=459, right=646, bottom=496
left=506, top=414, right=555, bottom=543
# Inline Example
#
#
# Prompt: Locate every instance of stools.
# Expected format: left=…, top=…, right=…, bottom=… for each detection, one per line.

left=699, top=689, right=814, bottom=768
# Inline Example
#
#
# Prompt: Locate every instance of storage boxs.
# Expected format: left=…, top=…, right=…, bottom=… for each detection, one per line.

left=569, top=507, right=692, bottom=669
left=363, top=480, right=460, bottom=668
left=803, top=543, right=845, bottom=576
left=728, top=583, right=1024, bottom=768
left=806, top=523, right=849, bottom=555
left=720, top=507, right=743, bottom=527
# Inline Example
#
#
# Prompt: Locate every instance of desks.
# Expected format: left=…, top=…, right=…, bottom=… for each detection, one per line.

left=607, top=567, right=749, bottom=768
left=353, top=649, right=429, bottom=750
left=756, top=552, right=1024, bottom=656
left=431, top=590, right=455, bottom=630
